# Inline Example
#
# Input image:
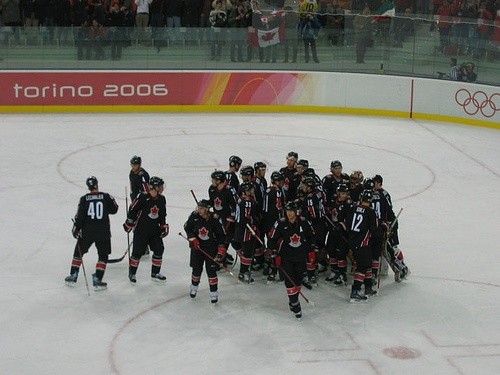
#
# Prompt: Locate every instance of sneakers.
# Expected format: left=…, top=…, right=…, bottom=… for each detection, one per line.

left=65, top=270, right=78, bottom=286
left=210, top=290, right=217, bottom=305
left=151, top=273, right=166, bottom=281
left=129, top=274, right=136, bottom=285
left=216, top=253, right=411, bottom=321
left=190, top=285, right=197, bottom=301
left=93, top=273, right=107, bottom=290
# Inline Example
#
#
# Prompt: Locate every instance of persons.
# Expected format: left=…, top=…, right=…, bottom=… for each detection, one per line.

left=208, top=151, right=411, bottom=321
left=123, top=176, right=169, bottom=286
left=183, top=199, right=228, bottom=306
left=128, top=156, right=150, bottom=259
left=64, top=176, right=118, bottom=291
left=18, top=0, right=500, bottom=84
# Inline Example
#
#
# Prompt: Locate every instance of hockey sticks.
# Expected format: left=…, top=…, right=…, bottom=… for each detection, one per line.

left=248, top=236, right=256, bottom=285
left=191, top=189, right=198, bottom=205
left=217, top=249, right=238, bottom=274
left=364, top=232, right=386, bottom=299
left=126, top=186, right=130, bottom=260
left=324, top=214, right=349, bottom=243
left=77, top=235, right=90, bottom=296
left=107, top=240, right=133, bottom=263
left=246, top=222, right=316, bottom=306
left=387, top=208, right=404, bottom=236
left=179, top=232, right=233, bottom=276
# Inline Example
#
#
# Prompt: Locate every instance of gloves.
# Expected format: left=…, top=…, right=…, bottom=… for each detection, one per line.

left=214, top=245, right=227, bottom=264
left=308, top=252, right=316, bottom=263
left=123, top=219, right=136, bottom=233
left=159, top=224, right=169, bottom=238
left=189, top=237, right=200, bottom=252
left=275, top=256, right=281, bottom=268
left=72, top=223, right=80, bottom=239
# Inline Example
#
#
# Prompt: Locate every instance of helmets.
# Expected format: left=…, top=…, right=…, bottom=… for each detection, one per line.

left=211, top=152, right=383, bottom=210
left=150, top=176, right=164, bottom=185
left=198, top=199, right=211, bottom=208
left=86, top=176, right=97, bottom=186
left=130, top=155, right=141, bottom=164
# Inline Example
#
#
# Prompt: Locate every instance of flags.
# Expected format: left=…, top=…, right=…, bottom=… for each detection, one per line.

left=370, top=1, right=395, bottom=25
left=247, top=23, right=286, bottom=48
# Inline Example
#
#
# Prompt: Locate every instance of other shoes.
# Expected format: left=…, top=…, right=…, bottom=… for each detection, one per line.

left=145, top=247, right=149, bottom=254
